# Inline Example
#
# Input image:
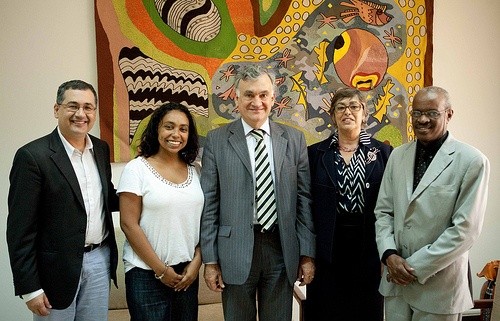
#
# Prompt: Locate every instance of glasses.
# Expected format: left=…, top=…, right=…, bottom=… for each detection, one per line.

left=408, top=108, right=447, bottom=119
left=334, top=104, right=362, bottom=114
left=58, top=103, right=97, bottom=113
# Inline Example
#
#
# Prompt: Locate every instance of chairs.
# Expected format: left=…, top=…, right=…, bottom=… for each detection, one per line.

left=462, top=261, right=500, bottom=321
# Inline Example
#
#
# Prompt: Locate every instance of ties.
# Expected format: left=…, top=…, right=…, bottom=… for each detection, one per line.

left=251, top=129, right=278, bottom=229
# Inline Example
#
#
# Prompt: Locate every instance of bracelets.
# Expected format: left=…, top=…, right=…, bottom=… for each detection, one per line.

left=155, top=264, right=169, bottom=280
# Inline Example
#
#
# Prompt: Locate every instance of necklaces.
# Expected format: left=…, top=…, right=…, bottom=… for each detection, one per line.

left=338, top=143, right=363, bottom=153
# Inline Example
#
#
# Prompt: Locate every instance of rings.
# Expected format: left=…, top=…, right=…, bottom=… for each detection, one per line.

left=312, top=276, right=314, bottom=278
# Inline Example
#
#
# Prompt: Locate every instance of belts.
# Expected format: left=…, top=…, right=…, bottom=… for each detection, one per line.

left=84, top=238, right=109, bottom=253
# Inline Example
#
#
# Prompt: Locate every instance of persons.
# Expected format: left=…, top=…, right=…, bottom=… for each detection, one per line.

left=116, top=102, right=205, bottom=321
left=306, top=86, right=395, bottom=321
left=201, top=62, right=316, bottom=321
left=6, top=79, right=120, bottom=321
left=374, top=86, right=490, bottom=321
